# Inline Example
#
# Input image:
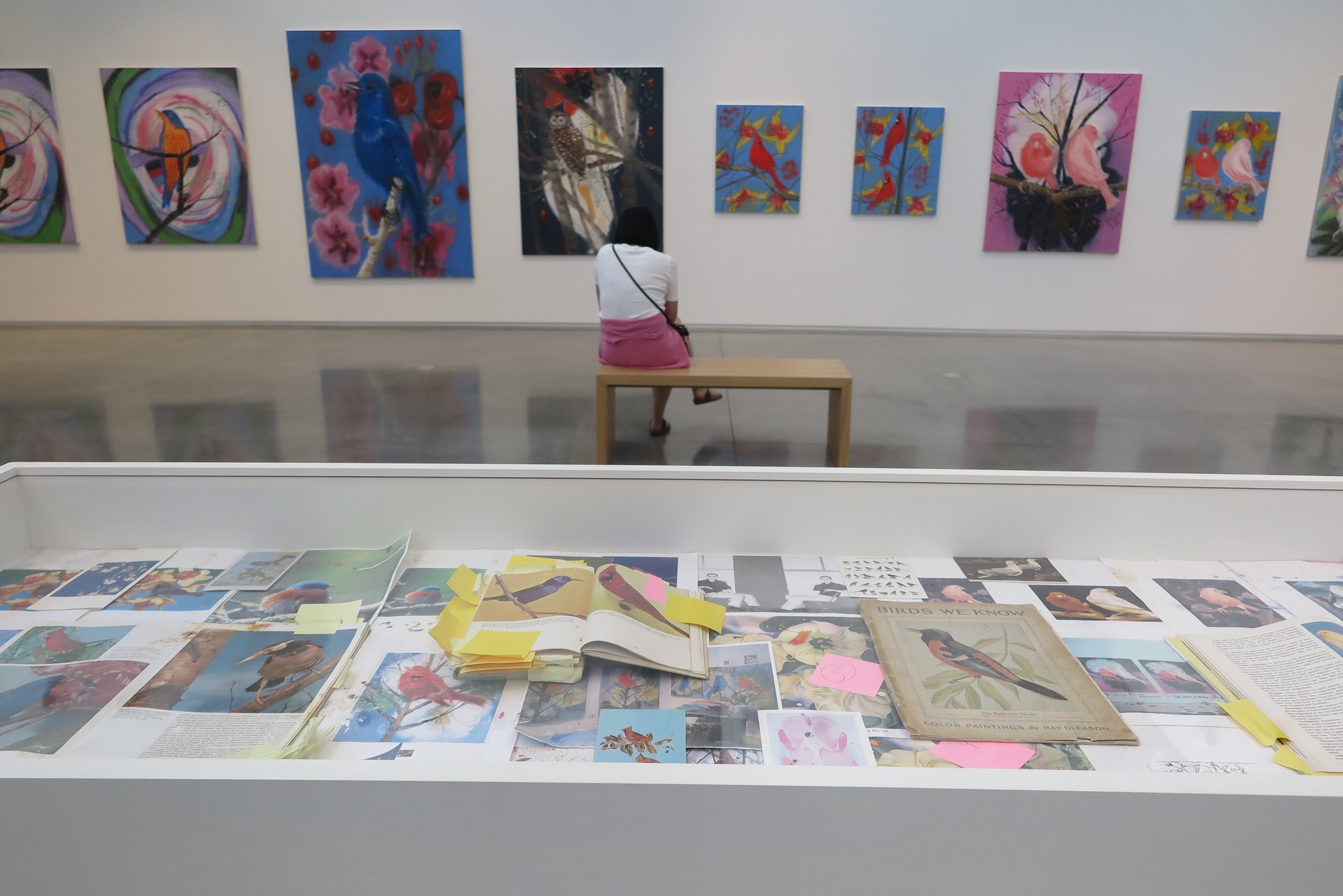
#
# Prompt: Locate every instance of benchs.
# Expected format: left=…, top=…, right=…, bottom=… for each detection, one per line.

left=596, top=357, right=853, bottom=468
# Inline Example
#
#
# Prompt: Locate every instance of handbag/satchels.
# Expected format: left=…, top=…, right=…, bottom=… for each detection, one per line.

left=668, top=322, right=694, bottom=357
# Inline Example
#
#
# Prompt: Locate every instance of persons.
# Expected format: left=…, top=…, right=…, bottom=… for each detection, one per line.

left=593, top=206, right=724, bottom=437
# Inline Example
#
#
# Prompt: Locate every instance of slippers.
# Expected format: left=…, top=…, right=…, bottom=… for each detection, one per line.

left=649, top=419, right=670, bottom=436
left=694, top=388, right=722, bottom=405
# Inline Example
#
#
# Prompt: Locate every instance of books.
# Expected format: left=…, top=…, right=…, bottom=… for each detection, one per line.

left=455, top=563, right=710, bottom=685
